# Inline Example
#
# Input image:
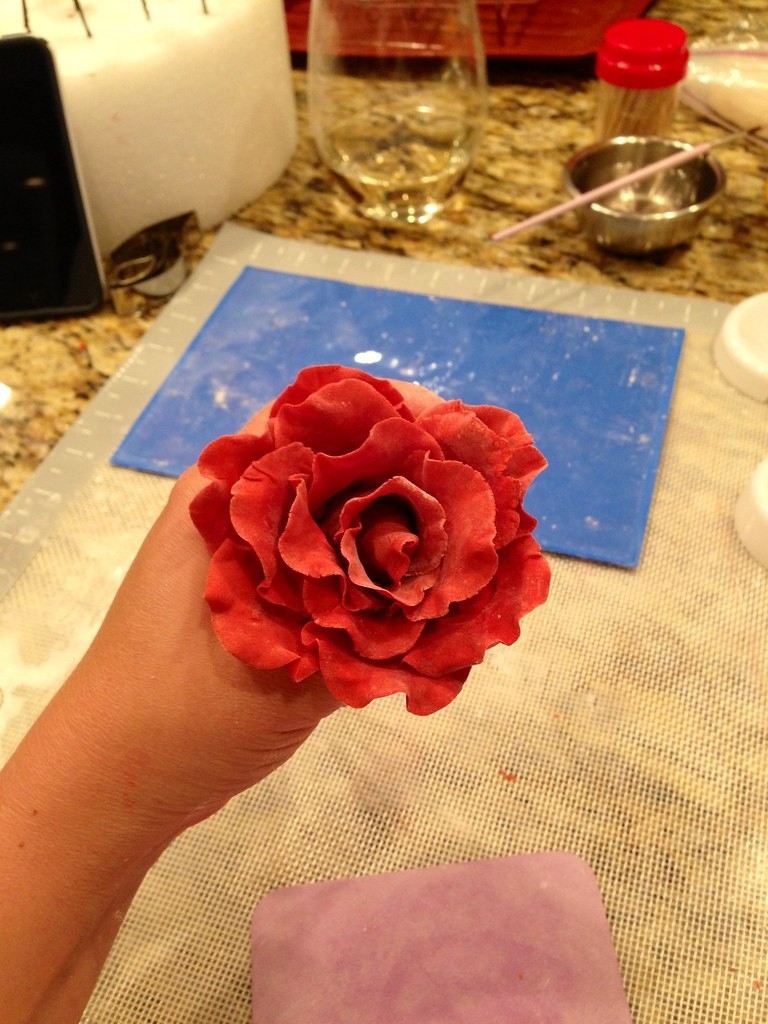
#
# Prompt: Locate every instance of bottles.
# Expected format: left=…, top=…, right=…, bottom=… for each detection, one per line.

left=594, top=17, right=690, bottom=141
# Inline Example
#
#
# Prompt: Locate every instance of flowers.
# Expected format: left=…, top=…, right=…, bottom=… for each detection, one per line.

left=190, top=364, right=551, bottom=717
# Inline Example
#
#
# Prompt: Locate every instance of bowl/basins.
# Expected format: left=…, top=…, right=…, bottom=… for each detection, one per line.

left=561, top=137, right=726, bottom=253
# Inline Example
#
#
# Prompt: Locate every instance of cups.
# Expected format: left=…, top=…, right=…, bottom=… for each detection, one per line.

left=307, top=0, right=487, bottom=227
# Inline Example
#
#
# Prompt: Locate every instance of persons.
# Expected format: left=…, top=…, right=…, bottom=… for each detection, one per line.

left=0, top=378, right=454, bottom=1023
